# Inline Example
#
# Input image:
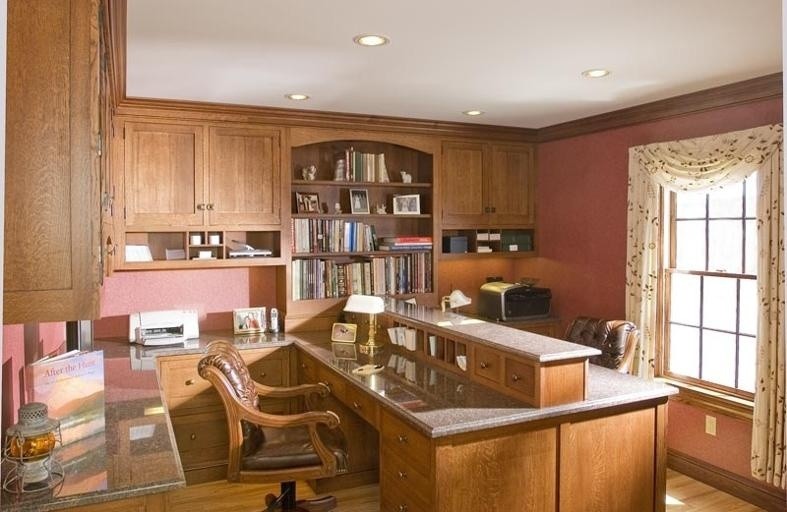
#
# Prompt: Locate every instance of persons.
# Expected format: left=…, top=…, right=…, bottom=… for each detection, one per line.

left=242, top=316, right=250, bottom=329
left=353, top=193, right=362, bottom=208
left=248, top=312, right=260, bottom=328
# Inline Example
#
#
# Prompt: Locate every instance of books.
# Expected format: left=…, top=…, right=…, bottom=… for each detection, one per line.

left=291, top=218, right=433, bottom=302
left=385, top=327, right=416, bottom=351
left=457, top=355, right=467, bottom=371
left=429, top=336, right=436, bottom=355
left=21, top=346, right=106, bottom=450
left=386, top=353, right=436, bottom=386
left=344, top=146, right=391, bottom=183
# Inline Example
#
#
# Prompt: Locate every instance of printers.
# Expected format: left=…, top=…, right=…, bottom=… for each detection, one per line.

left=128, top=310, right=200, bottom=346
left=479, top=280, right=552, bottom=322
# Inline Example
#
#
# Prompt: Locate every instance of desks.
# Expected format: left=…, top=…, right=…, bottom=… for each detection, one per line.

left=302, top=325, right=681, bottom=512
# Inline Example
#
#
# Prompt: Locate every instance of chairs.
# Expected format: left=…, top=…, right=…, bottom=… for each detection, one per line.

left=560, top=315, right=641, bottom=376
